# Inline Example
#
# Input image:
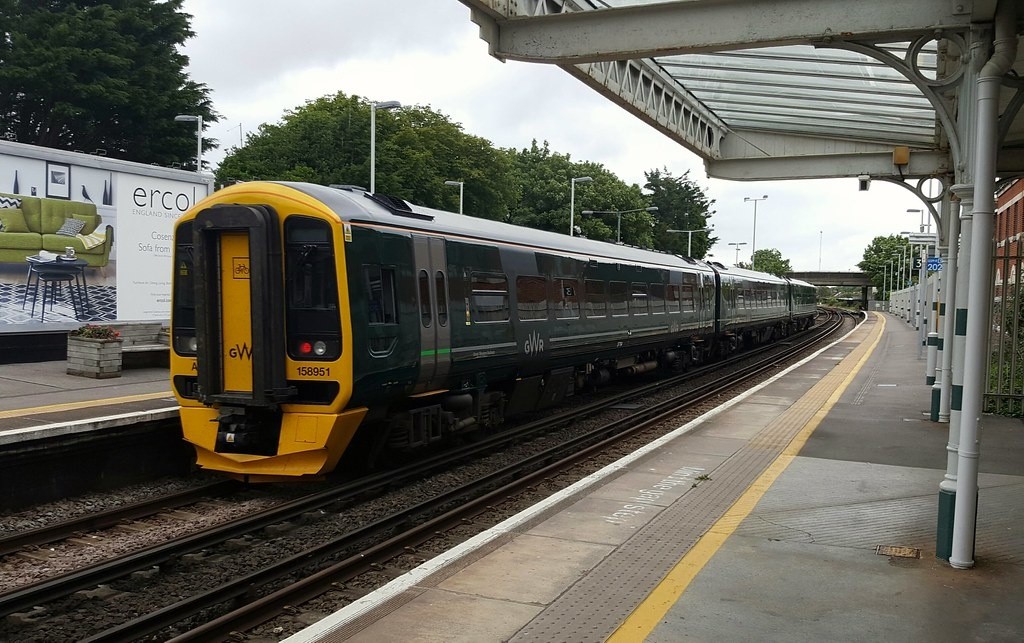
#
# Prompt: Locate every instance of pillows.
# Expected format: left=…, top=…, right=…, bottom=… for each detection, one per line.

left=0, top=207, right=30, bottom=233
left=72, top=213, right=96, bottom=236
left=56, top=217, right=87, bottom=237
left=0, top=195, right=22, bottom=231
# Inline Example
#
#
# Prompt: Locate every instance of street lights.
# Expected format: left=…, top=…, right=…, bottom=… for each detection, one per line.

left=729, top=243, right=747, bottom=264
left=891, top=253, right=900, bottom=316
left=371, top=102, right=403, bottom=192
left=666, top=229, right=715, bottom=257
left=879, top=265, right=885, bottom=311
left=900, top=231, right=913, bottom=323
left=895, top=246, right=906, bottom=318
left=570, top=177, right=593, bottom=236
left=444, top=181, right=464, bottom=214
left=885, top=260, right=893, bottom=314
left=582, top=207, right=659, bottom=244
left=907, top=209, right=924, bottom=359
left=174, top=114, right=203, bottom=170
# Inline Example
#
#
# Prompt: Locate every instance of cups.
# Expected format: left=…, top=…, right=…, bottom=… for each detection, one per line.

left=65, top=246, right=75, bottom=258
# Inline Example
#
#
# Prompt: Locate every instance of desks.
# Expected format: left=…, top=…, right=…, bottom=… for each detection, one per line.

left=22, top=253, right=89, bottom=323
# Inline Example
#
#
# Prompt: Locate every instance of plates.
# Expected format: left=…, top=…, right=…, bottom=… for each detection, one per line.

left=60, top=255, right=77, bottom=261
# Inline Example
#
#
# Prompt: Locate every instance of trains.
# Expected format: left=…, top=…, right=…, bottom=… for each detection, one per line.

left=169, top=179, right=819, bottom=484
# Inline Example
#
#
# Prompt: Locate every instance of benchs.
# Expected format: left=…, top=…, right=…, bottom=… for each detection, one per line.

left=0, top=192, right=115, bottom=277
left=99, top=322, right=170, bottom=371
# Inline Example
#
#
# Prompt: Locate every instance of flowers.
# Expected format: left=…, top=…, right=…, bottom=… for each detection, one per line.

left=79, top=322, right=118, bottom=340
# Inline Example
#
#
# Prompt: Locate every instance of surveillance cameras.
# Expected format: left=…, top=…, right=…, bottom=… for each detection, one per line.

left=576, top=228, right=581, bottom=234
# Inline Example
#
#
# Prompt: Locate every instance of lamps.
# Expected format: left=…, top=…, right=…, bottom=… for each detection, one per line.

left=857, top=174, right=871, bottom=191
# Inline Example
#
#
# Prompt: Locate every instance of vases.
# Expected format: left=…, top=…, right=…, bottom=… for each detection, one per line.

left=65, top=335, right=124, bottom=380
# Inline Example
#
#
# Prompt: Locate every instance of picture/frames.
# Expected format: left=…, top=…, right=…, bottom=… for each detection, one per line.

left=46, top=160, right=71, bottom=200
